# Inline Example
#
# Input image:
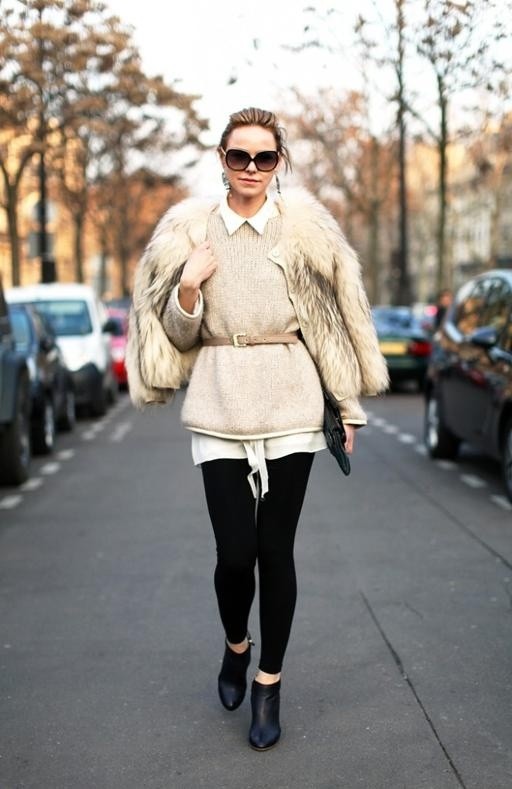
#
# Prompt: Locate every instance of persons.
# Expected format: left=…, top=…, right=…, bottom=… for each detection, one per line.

left=125, top=108, right=391, bottom=752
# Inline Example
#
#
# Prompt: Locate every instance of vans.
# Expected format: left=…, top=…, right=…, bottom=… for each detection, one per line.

left=1, top=280, right=122, bottom=419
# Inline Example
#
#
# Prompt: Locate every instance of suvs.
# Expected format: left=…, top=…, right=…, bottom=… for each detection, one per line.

left=0, top=279, right=40, bottom=492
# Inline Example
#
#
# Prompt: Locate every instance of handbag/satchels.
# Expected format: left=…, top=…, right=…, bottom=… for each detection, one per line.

left=323, top=394, right=350, bottom=475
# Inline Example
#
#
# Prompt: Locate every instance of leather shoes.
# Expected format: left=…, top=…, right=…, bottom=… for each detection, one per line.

left=219, top=631, right=250, bottom=710
left=249, top=678, right=280, bottom=751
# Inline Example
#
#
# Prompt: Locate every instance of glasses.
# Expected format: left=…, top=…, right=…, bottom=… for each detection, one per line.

left=220, top=148, right=279, bottom=172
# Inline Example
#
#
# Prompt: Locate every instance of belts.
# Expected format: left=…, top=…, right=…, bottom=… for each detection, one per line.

left=202, top=332, right=300, bottom=347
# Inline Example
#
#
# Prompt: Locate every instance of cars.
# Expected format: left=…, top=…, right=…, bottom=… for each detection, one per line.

left=105, top=305, right=132, bottom=387
left=422, top=266, right=512, bottom=505
left=6, top=302, right=82, bottom=456
left=364, top=305, right=433, bottom=391
